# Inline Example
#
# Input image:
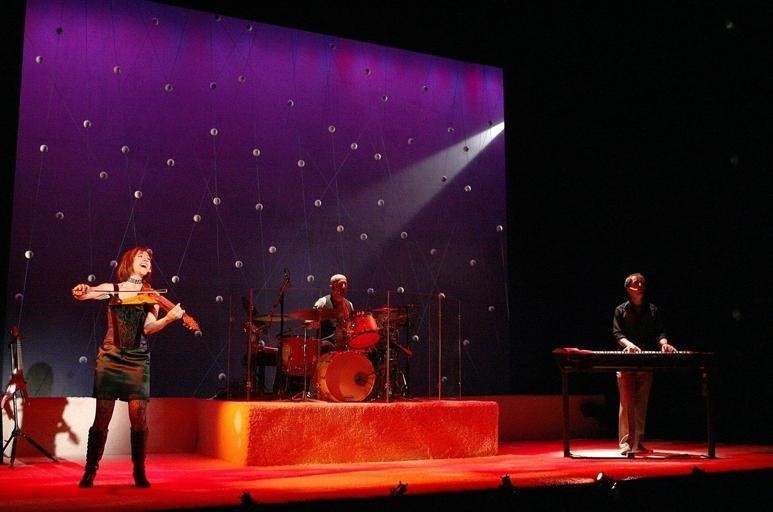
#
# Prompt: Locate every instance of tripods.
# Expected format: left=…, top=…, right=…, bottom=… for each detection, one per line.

left=256, top=282, right=329, bottom=403
left=3, top=271, right=59, bottom=469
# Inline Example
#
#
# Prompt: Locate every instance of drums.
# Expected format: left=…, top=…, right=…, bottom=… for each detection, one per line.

left=342, top=310, right=381, bottom=351
left=279, top=334, right=322, bottom=377
left=311, top=351, right=375, bottom=402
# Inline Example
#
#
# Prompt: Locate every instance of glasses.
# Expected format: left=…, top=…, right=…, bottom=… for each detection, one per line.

left=633, top=279, right=646, bottom=286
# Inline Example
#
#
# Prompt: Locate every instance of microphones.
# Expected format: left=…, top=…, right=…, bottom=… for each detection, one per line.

left=25, top=250, right=34, bottom=270
left=284, top=273, right=291, bottom=285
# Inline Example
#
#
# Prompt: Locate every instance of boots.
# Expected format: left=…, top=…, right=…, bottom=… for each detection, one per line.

left=130, top=426, right=149, bottom=487
left=80, top=428, right=107, bottom=487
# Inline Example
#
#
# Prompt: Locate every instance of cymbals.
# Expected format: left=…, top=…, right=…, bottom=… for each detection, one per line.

left=375, top=311, right=416, bottom=325
left=373, top=302, right=413, bottom=313
left=289, top=307, right=341, bottom=321
left=255, top=314, right=296, bottom=323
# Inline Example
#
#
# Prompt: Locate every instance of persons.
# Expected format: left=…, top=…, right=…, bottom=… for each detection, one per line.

left=308, top=273, right=355, bottom=397
left=612, top=272, right=679, bottom=455
left=72, top=246, right=185, bottom=488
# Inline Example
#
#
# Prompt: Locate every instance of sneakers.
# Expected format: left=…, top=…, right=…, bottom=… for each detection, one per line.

left=621, top=445, right=631, bottom=455
left=633, top=445, right=653, bottom=453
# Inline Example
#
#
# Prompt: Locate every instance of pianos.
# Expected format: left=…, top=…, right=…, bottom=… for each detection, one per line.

left=563, top=348, right=704, bottom=369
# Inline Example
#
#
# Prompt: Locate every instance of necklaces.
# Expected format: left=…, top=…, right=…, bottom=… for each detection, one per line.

left=127, top=277, right=144, bottom=284
